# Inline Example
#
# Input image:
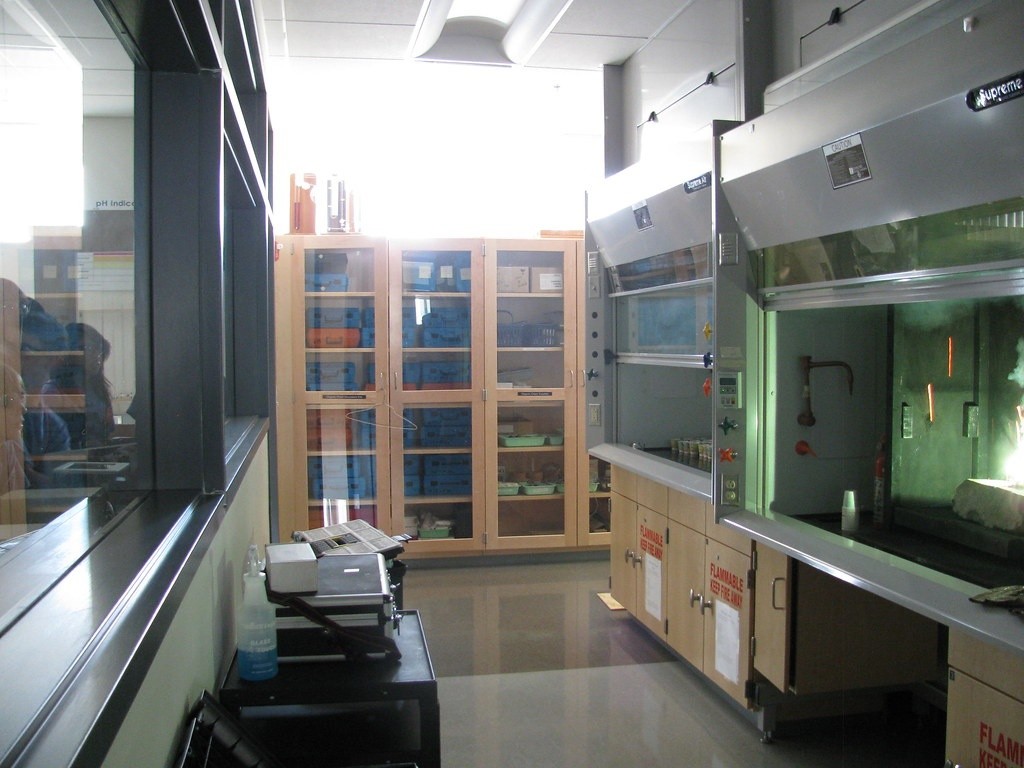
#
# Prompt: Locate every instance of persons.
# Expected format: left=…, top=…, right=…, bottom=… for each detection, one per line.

left=0, top=278, right=71, bottom=493
left=42, top=322, right=114, bottom=454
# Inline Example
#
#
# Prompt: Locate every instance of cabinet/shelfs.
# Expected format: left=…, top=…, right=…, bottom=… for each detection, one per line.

left=667, top=517, right=754, bottom=712
left=291, top=235, right=486, bottom=553
left=944, top=667, right=1024, bottom=768
left=609, top=490, right=670, bottom=647
left=1, top=231, right=90, bottom=546
left=484, top=239, right=611, bottom=551
left=753, top=540, right=800, bottom=698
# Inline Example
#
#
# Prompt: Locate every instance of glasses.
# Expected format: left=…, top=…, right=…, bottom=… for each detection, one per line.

left=75, top=346, right=104, bottom=358
left=0, top=392, right=25, bottom=409
left=20, top=345, right=52, bottom=359
left=0, top=305, right=29, bottom=319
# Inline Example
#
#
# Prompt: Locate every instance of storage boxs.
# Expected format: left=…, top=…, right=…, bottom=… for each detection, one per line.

left=272, top=552, right=401, bottom=663
left=495, top=264, right=608, bottom=535
left=290, top=518, right=408, bottom=612
left=33, top=250, right=84, bottom=294
left=305, top=249, right=472, bottom=541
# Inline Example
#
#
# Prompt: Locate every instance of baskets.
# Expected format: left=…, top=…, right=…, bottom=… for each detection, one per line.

left=498, top=308, right=525, bottom=347
left=524, top=323, right=559, bottom=347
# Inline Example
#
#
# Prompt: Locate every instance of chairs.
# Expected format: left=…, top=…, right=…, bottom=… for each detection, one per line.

left=172, top=688, right=426, bottom=768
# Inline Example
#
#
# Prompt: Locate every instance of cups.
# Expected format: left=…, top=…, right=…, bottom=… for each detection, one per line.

left=842, top=490, right=859, bottom=531
left=668, top=437, right=712, bottom=463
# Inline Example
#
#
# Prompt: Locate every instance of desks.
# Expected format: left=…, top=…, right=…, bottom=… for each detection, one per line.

left=219, top=611, right=441, bottom=768
